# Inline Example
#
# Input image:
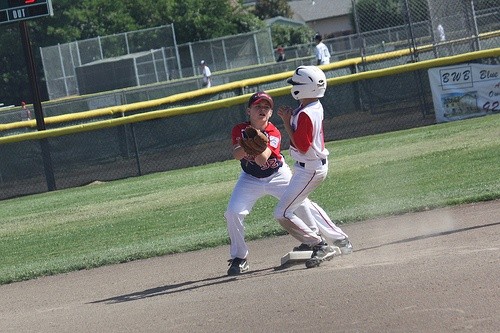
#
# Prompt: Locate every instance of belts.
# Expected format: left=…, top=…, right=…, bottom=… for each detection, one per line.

left=297, top=159, right=326, bottom=168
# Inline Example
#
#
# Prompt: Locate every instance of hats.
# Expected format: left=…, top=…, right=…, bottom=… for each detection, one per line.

left=200, top=59, right=205, bottom=65
left=314, top=35, right=322, bottom=40
left=248, top=92, right=275, bottom=109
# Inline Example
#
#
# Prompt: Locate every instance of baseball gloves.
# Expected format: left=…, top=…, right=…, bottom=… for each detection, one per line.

left=238, top=126, right=268, bottom=157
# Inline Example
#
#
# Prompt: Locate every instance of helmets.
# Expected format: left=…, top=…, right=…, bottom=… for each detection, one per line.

left=287, top=64, right=328, bottom=101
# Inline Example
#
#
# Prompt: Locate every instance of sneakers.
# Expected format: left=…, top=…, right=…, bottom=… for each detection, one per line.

left=292, top=243, right=314, bottom=252
left=226, top=257, right=249, bottom=276
left=334, top=238, right=354, bottom=254
left=305, top=242, right=336, bottom=268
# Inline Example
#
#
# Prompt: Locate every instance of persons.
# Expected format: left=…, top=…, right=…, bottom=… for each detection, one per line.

left=200, top=59, right=212, bottom=87
left=311, top=32, right=332, bottom=67
left=226, top=91, right=329, bottom=274
left=20, top=101, right=32, bottom=120
left=274, top=65, right=354, bottom=268
left=277, top=48, right=288, bottom=71
left=436, top=19, right=446, bottom=42
